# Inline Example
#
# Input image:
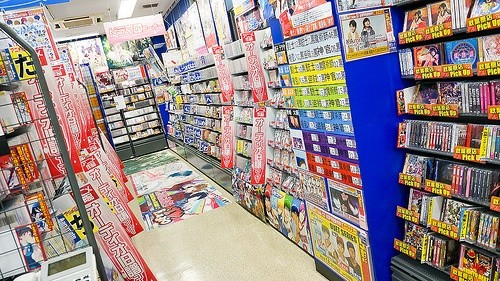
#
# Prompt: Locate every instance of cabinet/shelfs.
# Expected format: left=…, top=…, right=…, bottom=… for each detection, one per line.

left=390, top=0, right=500, bottom=281
left=92, top=28, right=313, bottom=258
